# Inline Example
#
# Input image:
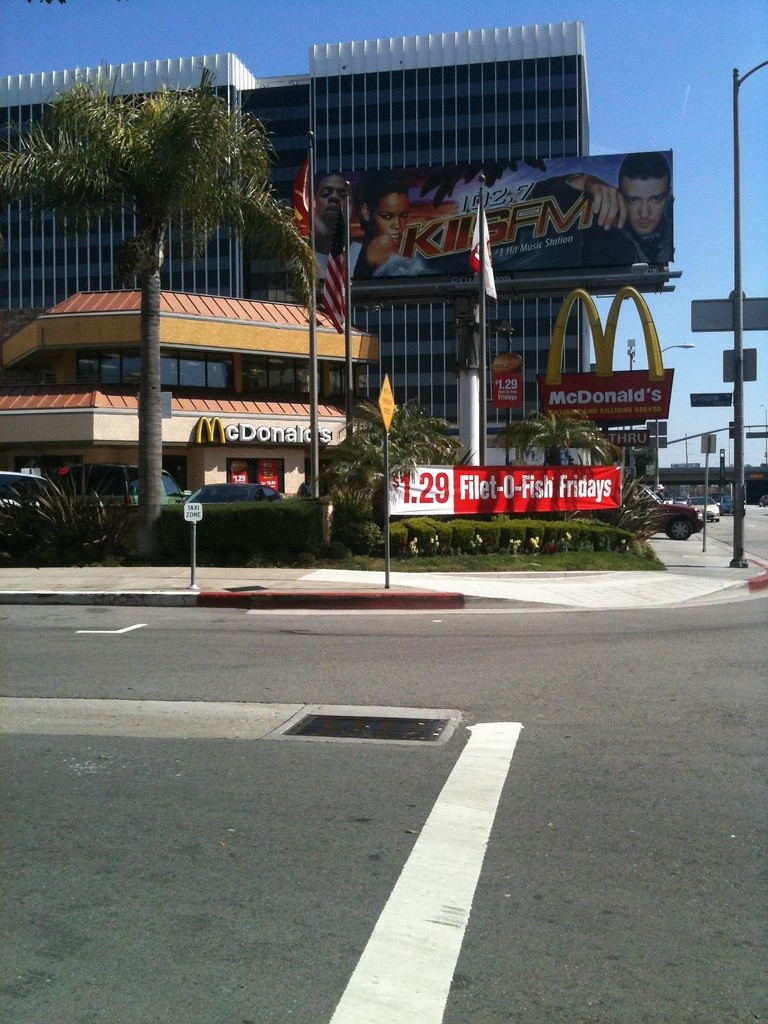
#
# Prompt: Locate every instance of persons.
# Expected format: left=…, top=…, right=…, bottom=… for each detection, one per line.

left=313, top=175, right=424, bottom=280
left=530, top=153, right=673, bottom=271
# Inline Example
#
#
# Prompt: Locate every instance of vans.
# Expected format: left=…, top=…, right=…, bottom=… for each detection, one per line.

left=719, top=498, right=746, bottom=516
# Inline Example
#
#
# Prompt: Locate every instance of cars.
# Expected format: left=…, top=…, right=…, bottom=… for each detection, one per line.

left=674, top=496, right=689, bottom=505
left=663, top=497, right=673, bottom=504
left=0, top=470, right=66, bottom=508
left=623, top=484, right=704, bottom=541
left=181, top=483, right=289, bottom=503
left=687, top=494, right=721, bottom=523
left=759, top=496, right=768, bottom=507
left=710, top=492, right=728, bottom=502
left=53, top=463, right=190, bottom=506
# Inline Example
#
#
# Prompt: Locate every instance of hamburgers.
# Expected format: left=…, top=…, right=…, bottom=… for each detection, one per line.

left=492, top=353, right=522, bottom=372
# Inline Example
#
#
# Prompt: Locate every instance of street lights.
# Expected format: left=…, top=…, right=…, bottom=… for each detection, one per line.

left=655, top=344, right=695, bottom=484
left=761, top=404, right=767, bottom=467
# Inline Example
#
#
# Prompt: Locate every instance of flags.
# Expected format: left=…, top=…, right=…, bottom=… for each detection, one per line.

left=319, top=246, right=347, bottom=334
left=469, top=203, right=499, bottom=300
left=292, top=161, right=312, bottom=241
